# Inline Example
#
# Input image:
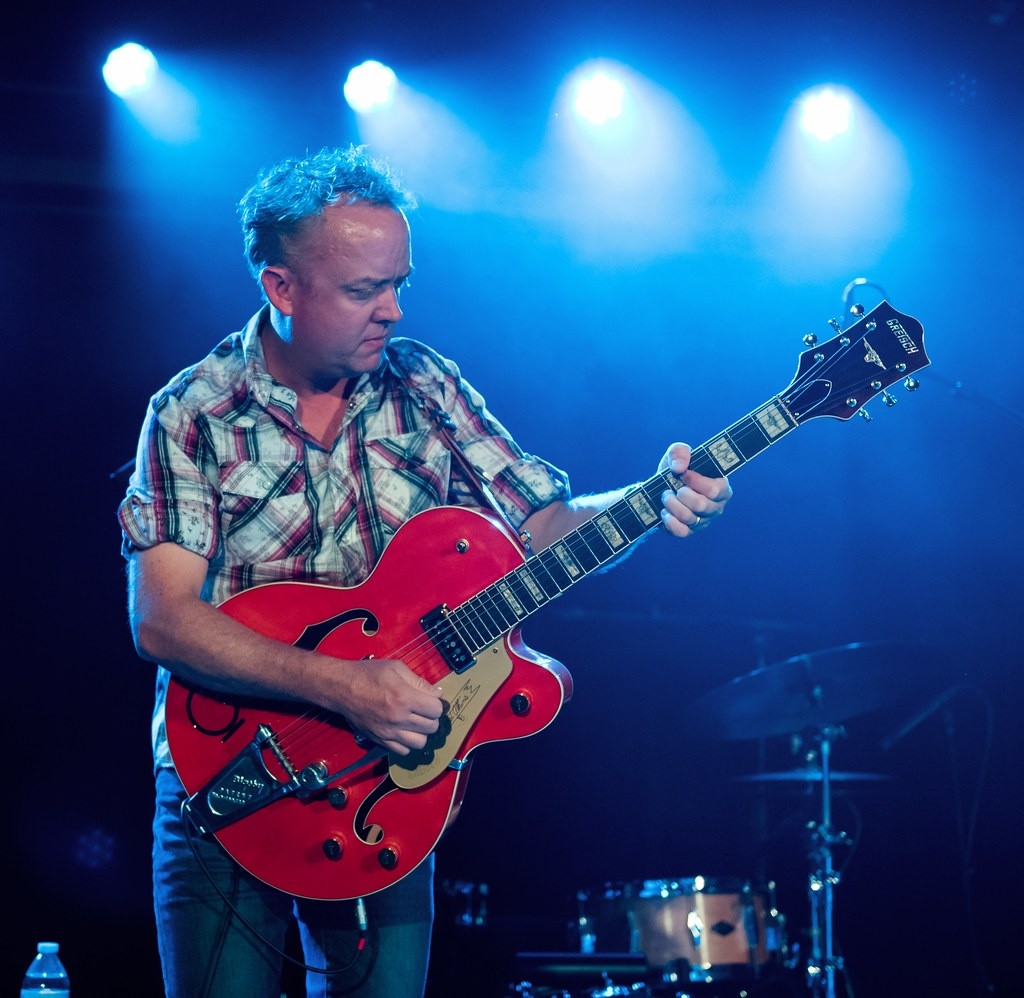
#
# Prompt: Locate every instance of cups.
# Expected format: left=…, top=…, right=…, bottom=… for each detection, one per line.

left=576, top=879, right=624, bottom=953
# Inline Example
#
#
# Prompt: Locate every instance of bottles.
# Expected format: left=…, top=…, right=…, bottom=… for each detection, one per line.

left=20, top=942, right=70, bottom=998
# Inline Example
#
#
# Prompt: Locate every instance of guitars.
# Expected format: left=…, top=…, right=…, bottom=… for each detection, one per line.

left=162, top=274, right=935, bottom=902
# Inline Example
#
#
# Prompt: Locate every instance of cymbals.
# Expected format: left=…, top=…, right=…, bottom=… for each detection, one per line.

left=744, top=764, right=896, bottom=787
left=684, top=637, right=899, bottom=746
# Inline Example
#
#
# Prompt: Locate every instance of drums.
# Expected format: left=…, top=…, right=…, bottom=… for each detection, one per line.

left=625, top=874, right=773, bottom=983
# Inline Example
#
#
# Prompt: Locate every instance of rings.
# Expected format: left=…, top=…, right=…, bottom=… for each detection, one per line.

left=688, top=516, right=700, bottom=529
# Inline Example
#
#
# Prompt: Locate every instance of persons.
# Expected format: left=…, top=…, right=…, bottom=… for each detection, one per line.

left=117, top=145, right=733, bottom=998
